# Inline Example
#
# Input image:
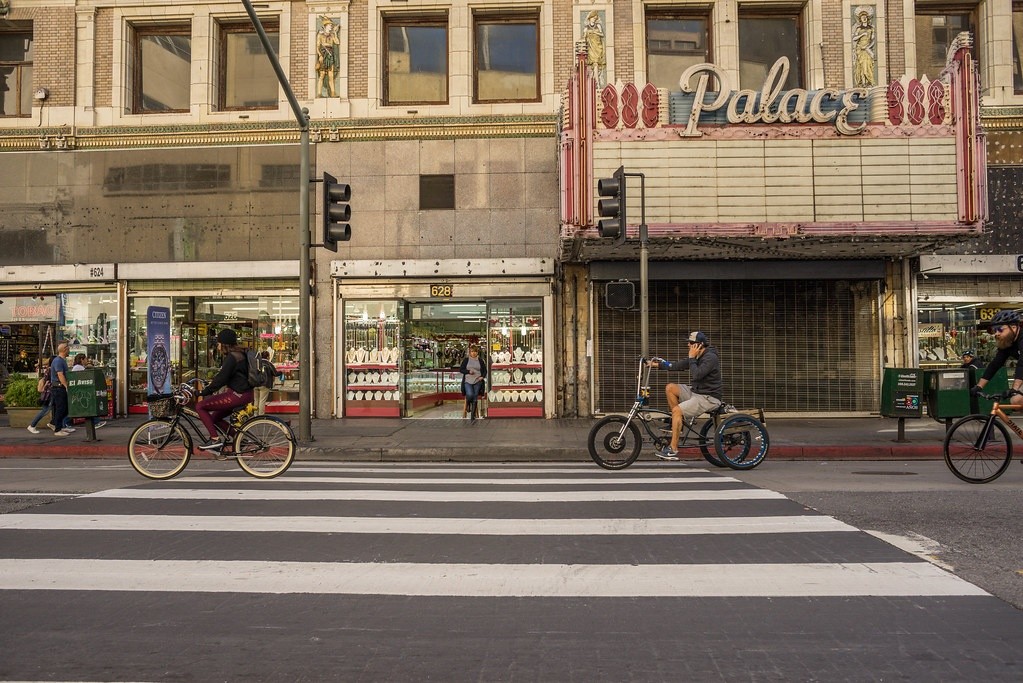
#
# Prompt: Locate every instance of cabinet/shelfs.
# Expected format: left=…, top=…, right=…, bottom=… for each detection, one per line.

left=266, top=362, right=300, bottom=414
left=18, top=342, right=46, bottom=354
left=346, top=363, right=542, bottom=417
left=126, top=364, right=147, bottom=413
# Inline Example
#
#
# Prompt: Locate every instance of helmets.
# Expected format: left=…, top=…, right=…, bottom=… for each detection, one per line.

left=991, top=310, right=1021, bottom=324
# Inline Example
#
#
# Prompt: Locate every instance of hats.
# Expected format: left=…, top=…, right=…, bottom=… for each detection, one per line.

left=217, top=329, right=237, bottom=344
left=962, top=351, right=974, bottom=357
left=686, top=331, right=706, bottom=345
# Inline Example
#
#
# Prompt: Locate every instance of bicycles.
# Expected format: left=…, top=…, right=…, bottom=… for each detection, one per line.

left=943, top=390, right=1023, bottom=484
left=127, top=378, right=298, bottom=480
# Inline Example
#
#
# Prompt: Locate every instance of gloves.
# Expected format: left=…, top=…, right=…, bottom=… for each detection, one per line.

left=971, top=385, right=983, bottom=397
left=1002, top=388, right=1016, bottom=400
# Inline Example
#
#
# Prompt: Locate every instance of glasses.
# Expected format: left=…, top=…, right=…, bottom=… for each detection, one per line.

left=993, top=326, right=1010, bottom=333
left=690, top=342, right=702, bottom=345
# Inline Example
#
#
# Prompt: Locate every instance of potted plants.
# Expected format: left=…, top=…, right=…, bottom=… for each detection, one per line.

left=4, top=372, right=51, bottom=429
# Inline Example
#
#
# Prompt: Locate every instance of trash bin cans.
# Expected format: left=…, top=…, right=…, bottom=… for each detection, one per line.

left=880, top=367, right=924, bottom=441
left=65, top=368, right=108, bottom=440
left=923, top=369, right=970, bottom=443
left=969, top=368, right=1012, bottom=442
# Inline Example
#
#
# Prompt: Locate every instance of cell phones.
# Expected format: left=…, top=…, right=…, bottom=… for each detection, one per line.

left=697, top=342, right=706, bottom=350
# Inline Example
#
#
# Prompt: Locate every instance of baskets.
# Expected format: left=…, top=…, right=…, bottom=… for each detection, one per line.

left=148, top=394, right=177, bottom=418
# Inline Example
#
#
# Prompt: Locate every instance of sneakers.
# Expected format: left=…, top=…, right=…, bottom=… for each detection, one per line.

left=198, top=438, right=223, bottom=449
left=654, top=448, right=679, bottom=460
left=241, top=440, right=251, bottom=451
left=658, top=425, right=683, bottom=434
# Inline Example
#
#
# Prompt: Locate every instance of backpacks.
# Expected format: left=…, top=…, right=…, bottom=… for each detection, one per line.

left=227, top=348, right=264, bottom=387
left=37, top=377, right=49, bottom=392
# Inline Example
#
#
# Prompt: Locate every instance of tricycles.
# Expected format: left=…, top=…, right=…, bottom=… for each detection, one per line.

left=587, top=353, right=770, bottom=471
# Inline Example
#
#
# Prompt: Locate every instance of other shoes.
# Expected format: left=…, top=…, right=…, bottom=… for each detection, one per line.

left=27, top=425, right=40, bottom=433
left=471, top=413, right=475, bottom=425
left=466, top=402, right=470, bottom=413
left=47, top=423, right=56, bottom=430
left=54, top=430, right=69, bottom=436
left=62, top=428, right=76, bottom=432
left=95, top=421, right=106, bottom=428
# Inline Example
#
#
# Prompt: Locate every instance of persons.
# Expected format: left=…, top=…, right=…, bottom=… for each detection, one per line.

left=13, top=352, right=28, bottom=373
left=960, top=351, right=983, bottom=376
left=459, top=344, right=487, bottom=425
left=61, top=353, right=107, bottom=432
left=50, top=342, right=75, bottom=436
left=194, top=329, right=254, bottom=456
left=27, top=356, right=58, bottom=433
left=253, top=351, right=282, bottom=417
left=644, top=331, right=722, bottom=460
left=971, top=310, right=1023, bottom=463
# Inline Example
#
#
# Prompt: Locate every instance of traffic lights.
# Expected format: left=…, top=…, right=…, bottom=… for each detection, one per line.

left=323, top=171, right=352, bottom=252
left=597, top=164, right=626, bottom=249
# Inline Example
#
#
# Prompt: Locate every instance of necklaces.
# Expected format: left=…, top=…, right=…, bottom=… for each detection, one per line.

left=516, top=351, right=522, bottom=357
left=515, top=372, right=521, bottom=379
left=348, top=349, right=398, bottom=362
